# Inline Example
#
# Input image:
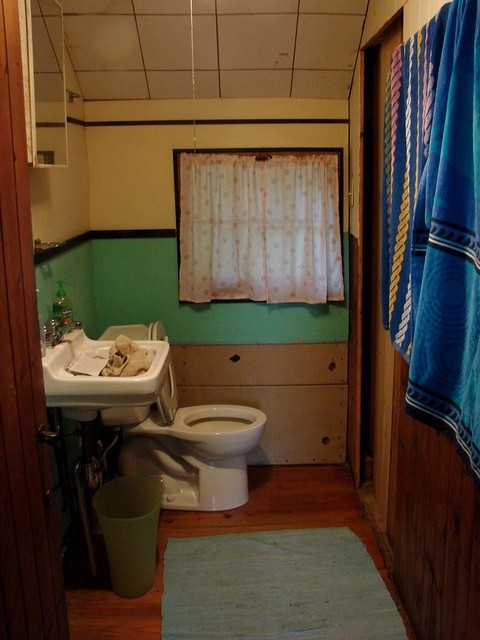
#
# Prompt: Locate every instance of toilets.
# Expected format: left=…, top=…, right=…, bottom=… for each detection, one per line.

left=98, top=321, right=267, bottom=513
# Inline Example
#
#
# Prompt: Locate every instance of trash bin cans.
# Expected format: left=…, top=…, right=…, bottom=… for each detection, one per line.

left=92, top=476, right=161, bottom=599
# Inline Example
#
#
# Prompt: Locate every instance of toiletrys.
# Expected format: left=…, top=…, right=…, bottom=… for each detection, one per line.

left=54, top=281, right=73, bottom=325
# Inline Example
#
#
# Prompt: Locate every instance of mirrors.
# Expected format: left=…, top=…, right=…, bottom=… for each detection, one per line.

left=18, top=0, right=68, bottom=171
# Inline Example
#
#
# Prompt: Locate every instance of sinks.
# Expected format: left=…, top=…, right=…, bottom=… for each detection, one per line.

left=42, top=329, right=169, bottom=393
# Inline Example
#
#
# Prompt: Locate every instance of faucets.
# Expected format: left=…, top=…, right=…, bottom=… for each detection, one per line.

left=49, top=320, right=82, bottom=346
